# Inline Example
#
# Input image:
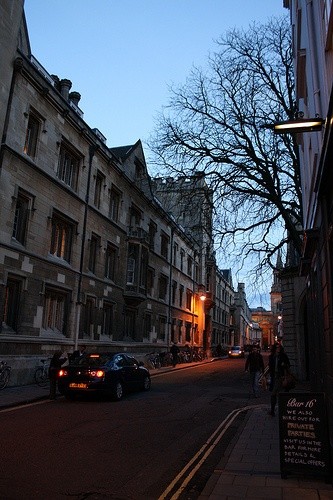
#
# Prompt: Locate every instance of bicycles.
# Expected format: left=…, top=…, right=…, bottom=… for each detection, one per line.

left=144, top=350, right=174, bottom=367
left=34, top=358, right=53, bottom=387
left=0, top=360, right=13, bottom=393
left=178, top=348, right=206, bottom=362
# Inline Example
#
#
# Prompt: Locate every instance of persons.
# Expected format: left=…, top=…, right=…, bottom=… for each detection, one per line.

left=185, top=342, right=192, bottom=356
left=216, top=343, right=222, bottom=357
left=170, top=342, right=180, bottom=368
left=158, top=346, right=167, bottom=358
left=244, top=346, right=265, bottom=398
left=71, top=346, right=87, bottom=361
left=263, top=343, right=290, bottom=417
left=47, top=352, right=70, bottom=400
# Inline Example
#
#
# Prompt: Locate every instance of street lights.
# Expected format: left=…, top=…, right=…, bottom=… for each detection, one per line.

left=191, top=289, right=206, bottom=348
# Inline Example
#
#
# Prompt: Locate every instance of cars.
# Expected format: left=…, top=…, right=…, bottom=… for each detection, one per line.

left=227, top=346, right=245, bottom=359
left=56, top=353, right=152, bottom=400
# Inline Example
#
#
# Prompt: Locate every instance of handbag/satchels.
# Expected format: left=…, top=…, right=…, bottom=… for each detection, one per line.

left=281, top=373, right=296, bottom=392
left=261, top=376, right=267, bottom=390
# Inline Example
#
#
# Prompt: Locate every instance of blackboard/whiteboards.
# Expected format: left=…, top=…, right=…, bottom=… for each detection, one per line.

left=277, top=391, right=331, bottom=473
